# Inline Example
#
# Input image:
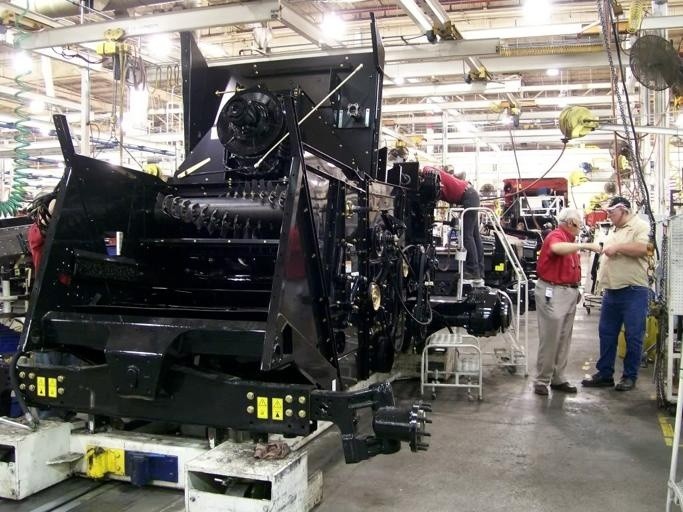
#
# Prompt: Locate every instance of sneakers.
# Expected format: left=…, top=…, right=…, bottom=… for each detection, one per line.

left=473, top=277, right=486, bottom=288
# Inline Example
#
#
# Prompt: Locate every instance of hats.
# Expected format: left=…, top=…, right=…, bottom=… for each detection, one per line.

left=606, top=196, right=632, bottom=213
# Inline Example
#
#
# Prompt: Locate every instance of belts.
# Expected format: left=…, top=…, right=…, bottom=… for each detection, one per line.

left=538, top=276, right=580, bottom=288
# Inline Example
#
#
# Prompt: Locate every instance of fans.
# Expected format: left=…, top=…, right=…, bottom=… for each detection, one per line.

left=628, top=34, right=683, bottom=96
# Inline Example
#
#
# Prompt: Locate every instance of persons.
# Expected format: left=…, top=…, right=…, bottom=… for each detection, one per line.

left=532, top=206, right=603, bottom=395
left=28, top=187, right=63, bottom=281
left=581, top=198, right=651, bottom=391
left=417, top=166, right=486, bottom=280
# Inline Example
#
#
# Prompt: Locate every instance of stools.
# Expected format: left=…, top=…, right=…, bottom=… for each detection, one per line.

left=420, top=332, right=484, bottom=402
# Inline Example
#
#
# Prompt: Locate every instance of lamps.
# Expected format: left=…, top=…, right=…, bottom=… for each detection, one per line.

left=556, top=105, right=683, bottom=146
left=432, top=21, right=492, bottom=81
left=500, top=90, right=523, bottom=122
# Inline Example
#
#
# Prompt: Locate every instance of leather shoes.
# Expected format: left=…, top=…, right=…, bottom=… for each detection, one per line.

left=534, top=383, right=548, bottom=395
left=615, top=374, right=637, bottom=391
left=581, top=372, right=615, bottom=387
left=549, top=381, right=578, bottom=394
